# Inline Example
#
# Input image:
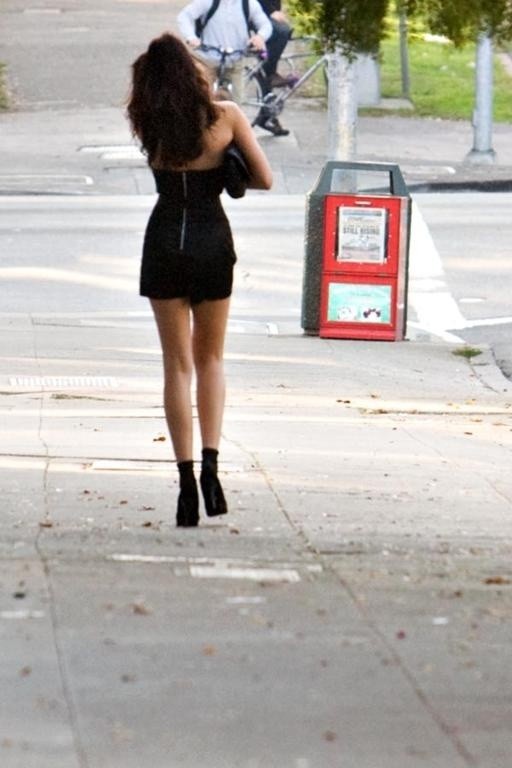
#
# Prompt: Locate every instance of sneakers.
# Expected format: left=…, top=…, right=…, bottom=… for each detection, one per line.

left=270, top=74, right=289, bottom=87
left=261, top=117, right=289, bottom=136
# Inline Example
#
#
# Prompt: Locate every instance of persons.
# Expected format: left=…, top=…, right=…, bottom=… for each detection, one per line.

left=126, top=33, right=272, bottom=528
left=174, top=0, right=301, bottom=137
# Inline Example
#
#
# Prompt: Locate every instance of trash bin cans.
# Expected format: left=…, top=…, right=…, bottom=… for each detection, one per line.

left=301, top=161, right=413, bottom=341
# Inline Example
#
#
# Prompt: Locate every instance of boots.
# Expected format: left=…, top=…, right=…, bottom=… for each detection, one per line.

left=200, top=444, right=229, bottom=518
left=172, top=461, right=201, bottom=531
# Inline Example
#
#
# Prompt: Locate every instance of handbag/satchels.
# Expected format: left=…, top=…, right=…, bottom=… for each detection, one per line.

left=222, top=140, right=252, bottom=201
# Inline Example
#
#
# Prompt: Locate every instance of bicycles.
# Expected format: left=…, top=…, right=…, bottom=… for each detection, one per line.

left=187, top=28, right=328, bottom=127
